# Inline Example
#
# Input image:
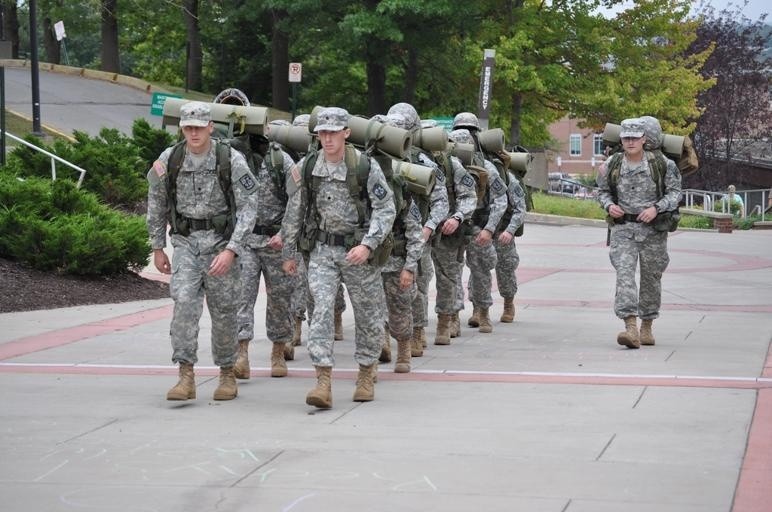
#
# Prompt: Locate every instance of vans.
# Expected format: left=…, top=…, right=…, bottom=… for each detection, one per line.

left=548, top=172, right=579, bottom=194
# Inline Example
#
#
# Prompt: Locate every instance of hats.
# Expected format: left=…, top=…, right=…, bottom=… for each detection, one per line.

left=294, top=114, right=311, bottom=127
left=620, top=118, right=648, bottom=140
left=179, top=102, right=213, bottom=128
left=313, top=107, right=349, bottom=132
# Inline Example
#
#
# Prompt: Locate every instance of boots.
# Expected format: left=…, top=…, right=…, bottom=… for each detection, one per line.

left=618, top=316, right=641, bottom=350
left=640, top=320, right=654, bottom=346
left=213, top=368, right=239, bottom=401
left=234, top=340, right=251, bottom=380
left=272, top=342, right=289, bottom=378
left=306, top=297, right=515, bottom=411
left=167, top=364, right=197, bottom=402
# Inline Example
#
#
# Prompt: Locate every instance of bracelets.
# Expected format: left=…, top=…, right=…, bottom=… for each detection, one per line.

left=653, top=203, right=661, bottom=213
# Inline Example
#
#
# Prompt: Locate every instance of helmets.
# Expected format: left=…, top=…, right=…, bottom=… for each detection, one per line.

left=388, top=103, right=421, bottom=131
left=453, top=112, right=482, bottom=132
left=214, top=88, right=251, bottom=108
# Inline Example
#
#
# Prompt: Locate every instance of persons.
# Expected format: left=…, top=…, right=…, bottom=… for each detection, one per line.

left=596, top=118, right=683, bottom=349
left=146, top=101, right=525, bottom=408
left=721, top=184, right=744, bottom=218
